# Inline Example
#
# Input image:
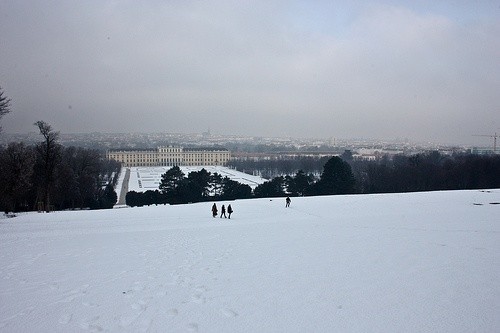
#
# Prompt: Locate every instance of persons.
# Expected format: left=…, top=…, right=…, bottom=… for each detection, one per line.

left=220, top=204, right=228, bottom=218
left=212, top=203, right=218, bottom=217
left=227, top=204, right=233, bottom=219
left=285, top=196, right=291, bottom=207
